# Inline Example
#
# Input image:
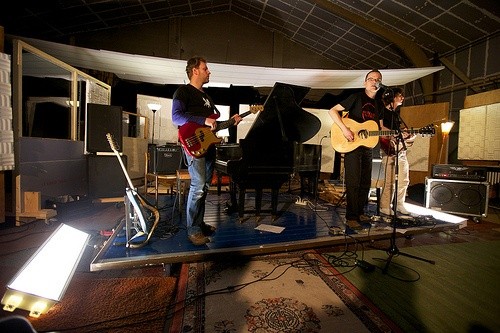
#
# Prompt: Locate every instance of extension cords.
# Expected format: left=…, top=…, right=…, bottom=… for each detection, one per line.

left=295, top=202, right=307, bottom=205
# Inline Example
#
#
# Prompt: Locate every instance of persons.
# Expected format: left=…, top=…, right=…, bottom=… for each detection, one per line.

left=170, top=55, right=241, bottom=245
left=328, top=66, right=400, bottom=231
left=375, top=86, right=415, bottom=218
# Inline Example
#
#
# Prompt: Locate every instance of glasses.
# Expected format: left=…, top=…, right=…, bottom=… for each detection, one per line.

left=366, top=77, right=382, bottom=82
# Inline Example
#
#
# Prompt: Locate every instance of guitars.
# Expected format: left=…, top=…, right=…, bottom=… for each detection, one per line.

left=329, top=118, right=434, bottom=155
left=105, top=133, right=159, bottom=247
left=179, top=105, right=264, bottom=158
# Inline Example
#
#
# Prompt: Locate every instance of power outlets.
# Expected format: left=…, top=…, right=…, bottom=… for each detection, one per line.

left=318, top=134, right=330, bottom=147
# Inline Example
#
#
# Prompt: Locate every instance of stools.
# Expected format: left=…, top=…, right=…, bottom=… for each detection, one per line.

left=175, top=169, right=191, bottom=212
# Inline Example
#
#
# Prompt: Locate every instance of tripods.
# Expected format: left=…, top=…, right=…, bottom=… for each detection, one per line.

left=365, top=97, right=435, bottom=274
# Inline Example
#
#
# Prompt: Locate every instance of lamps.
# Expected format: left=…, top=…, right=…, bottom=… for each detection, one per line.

left=148, top=103, right=162, bottom=143
left=0, top=223, right=92, bottom=319
left=438, top=121, right=455, bottom=164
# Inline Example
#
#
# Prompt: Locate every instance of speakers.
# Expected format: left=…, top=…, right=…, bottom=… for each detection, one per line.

left=88, top=102, right=188, bottom=198
left=424, top=177, right=488, bottom=217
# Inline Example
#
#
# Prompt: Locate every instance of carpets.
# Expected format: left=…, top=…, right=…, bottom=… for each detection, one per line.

left=168, top=248, right=403, bottom=333
left=0, top=202, right=177, bottom=333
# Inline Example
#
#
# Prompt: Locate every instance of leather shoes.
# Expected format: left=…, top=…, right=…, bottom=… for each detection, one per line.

left=198, top=222, right=216, bottom=232
left=188, top=231, right=210, bottom=245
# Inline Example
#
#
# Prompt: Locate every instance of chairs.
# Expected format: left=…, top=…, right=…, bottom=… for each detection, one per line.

left=144, top=153, right=177, bottom=208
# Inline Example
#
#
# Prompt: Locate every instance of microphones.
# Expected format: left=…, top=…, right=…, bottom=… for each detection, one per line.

left=376, top=82, right=392, bottom=93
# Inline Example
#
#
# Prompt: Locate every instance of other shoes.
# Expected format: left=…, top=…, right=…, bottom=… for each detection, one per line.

left=396, top=205, right=411, bottom=214
left=380, top=207, right=394, bottom=215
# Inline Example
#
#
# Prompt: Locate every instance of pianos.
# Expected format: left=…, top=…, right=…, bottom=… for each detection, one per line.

left=213, top=82, right=321, bottom=223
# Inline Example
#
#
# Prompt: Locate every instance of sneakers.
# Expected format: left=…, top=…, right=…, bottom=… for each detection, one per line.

left=358, top=214, right=372, bottom=223
left=346, top=218, right=361, bottom=229
